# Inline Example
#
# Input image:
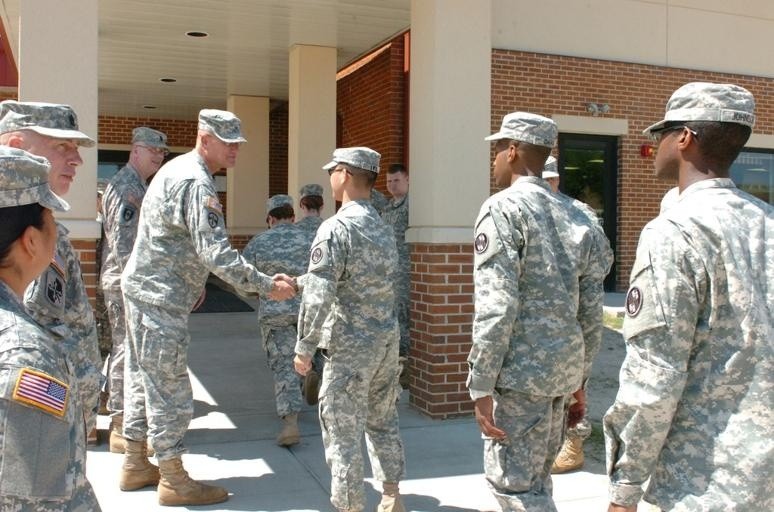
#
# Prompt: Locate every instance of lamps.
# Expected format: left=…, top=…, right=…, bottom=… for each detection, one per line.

left=586, top=103, right=610, bottom=116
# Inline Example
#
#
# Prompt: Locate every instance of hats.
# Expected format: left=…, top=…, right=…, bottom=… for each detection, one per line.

left=198, top=109, right=247, bottom=143
left=0, top=145, right=71, bottom=211
left=642, top=82, right=755, bottom=136
left=485, top=112, right=559, bottom=178
left=299, top=184, right=323, bottom=207
left=0, top=101, right=96, bottom=147
left=131, top=127, right=169, bottom=152
left=264, top=195, right=294, bottom=222
left=322, top=146, right=381, bottom=174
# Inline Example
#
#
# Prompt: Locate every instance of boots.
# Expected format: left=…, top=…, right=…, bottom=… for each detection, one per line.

left=109, top=415, right=228, bottom=505
left=277, top=411, right=300, bottom=445
left=303, top=372, right=320, bottom=406
left=376, top=483, right=405, bottom=512
left=552, top=436, right=584, bottom=474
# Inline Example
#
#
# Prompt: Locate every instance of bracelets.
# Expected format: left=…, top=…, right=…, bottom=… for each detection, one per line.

left=468, top=387, right=491, bottom=400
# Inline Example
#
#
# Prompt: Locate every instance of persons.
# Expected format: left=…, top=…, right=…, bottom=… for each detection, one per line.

left=540, top=155, right=615, bottom=474
left=466, top=112, right=609, bottom=511
left=0, top=145, right=103, bottom=512
left=0, top=99, right=102, bottom=438
left=602, top=81, right=772, bottom=510
left=377, top=163, right=410, bottom=389
left=119, top=108, right=294, bottom=506
left=268, top=147, right=406, bottom=511
left=293, top=184, right=324, bottom=405
left=234, top=194, right=319, bottom=446
left=96, top=177, right=114, bottom=413
left=101, top=125, right=169, bottom=454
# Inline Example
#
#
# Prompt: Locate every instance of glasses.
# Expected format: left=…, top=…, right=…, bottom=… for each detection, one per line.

left=650, top=125, right=698, bottom=142
left=328, top=169, right=354, bottom=176
left=136, top=144, right=169, bottom=156
